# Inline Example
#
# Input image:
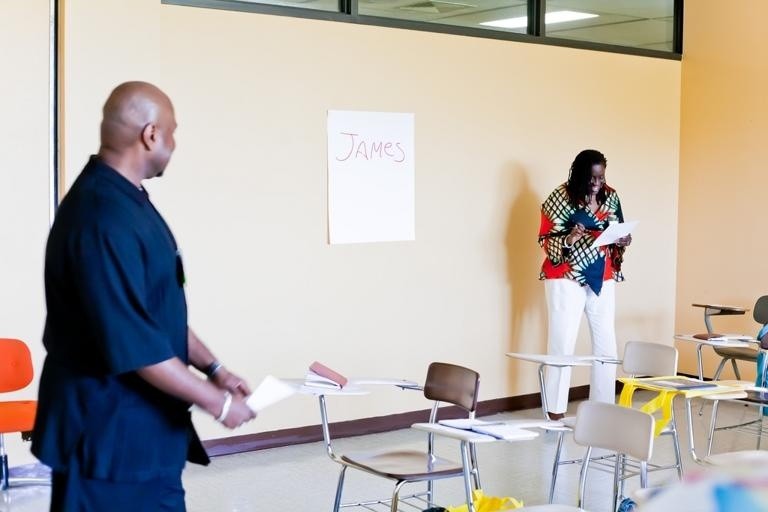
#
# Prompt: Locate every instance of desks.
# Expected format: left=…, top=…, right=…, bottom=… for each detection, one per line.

left=675, top=303, right=766, bottom=481
left=620, top=374, right=756, bottom=402
left=411, top=417, right=563, bottom=448
left=274, top=375, right=418, bottom=396
left=505, top=350, right=622, bottom=369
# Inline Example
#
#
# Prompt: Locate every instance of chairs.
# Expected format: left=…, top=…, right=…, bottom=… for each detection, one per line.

left=547, top=340, right=683, bottom=510
left=334, top=361, right=481, bottom=511
left=461, top=400, right=656, bottom=511
left=0, top=336, right=67, bottom=494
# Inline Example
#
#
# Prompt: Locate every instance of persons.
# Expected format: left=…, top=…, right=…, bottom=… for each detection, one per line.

left=538, top=150, right=632, bottom=420
left=30, top=81, right=258, bottom=512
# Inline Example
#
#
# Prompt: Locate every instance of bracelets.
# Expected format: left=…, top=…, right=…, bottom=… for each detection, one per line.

left=564, top=234, right=573, bottom=248
left=215, top=392, right=231, bottom=422
left=209, top=361, right=222, bottom=375
